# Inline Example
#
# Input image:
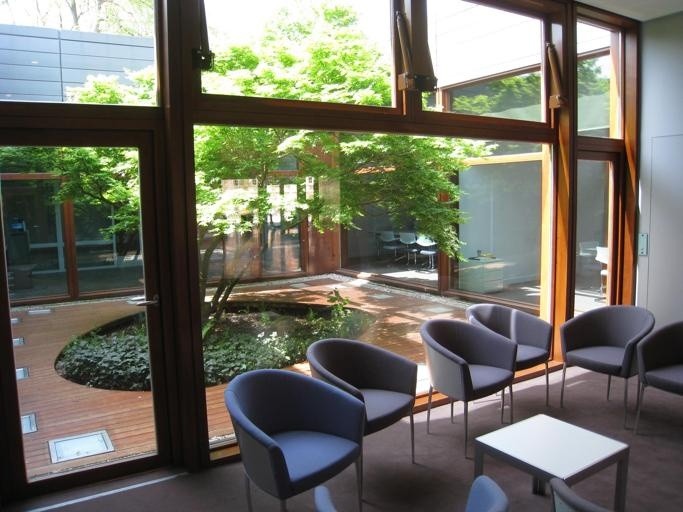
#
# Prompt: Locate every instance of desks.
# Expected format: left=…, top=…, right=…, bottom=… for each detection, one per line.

left=472, top=412, right=629, bottom=512
left=7, top=264, right=38, bottom=289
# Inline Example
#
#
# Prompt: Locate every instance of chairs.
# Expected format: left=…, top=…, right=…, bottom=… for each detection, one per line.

left=560, top=305, right=656, bottom=427
left=467, top=301, right=554, bottom=422
left=549, top=476, right=607, bottom=510
left=227, top=368, right=365, bottom=510
left=467, top=474, right=509, bottom=511
left=313, top=484, right=336, bottom=511
left=375, top=227, right=439, bottom=270
left=421, top=317, right=518, bottom=458
left=632, top=321, right=683, bottom=436
left=306, top=338, right=418, bottom=502
left=578, top=240, right=609, bottom=296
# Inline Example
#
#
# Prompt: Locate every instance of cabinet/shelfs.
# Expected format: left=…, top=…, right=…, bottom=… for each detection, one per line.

left=460, top=259, right=505, bottom=294
